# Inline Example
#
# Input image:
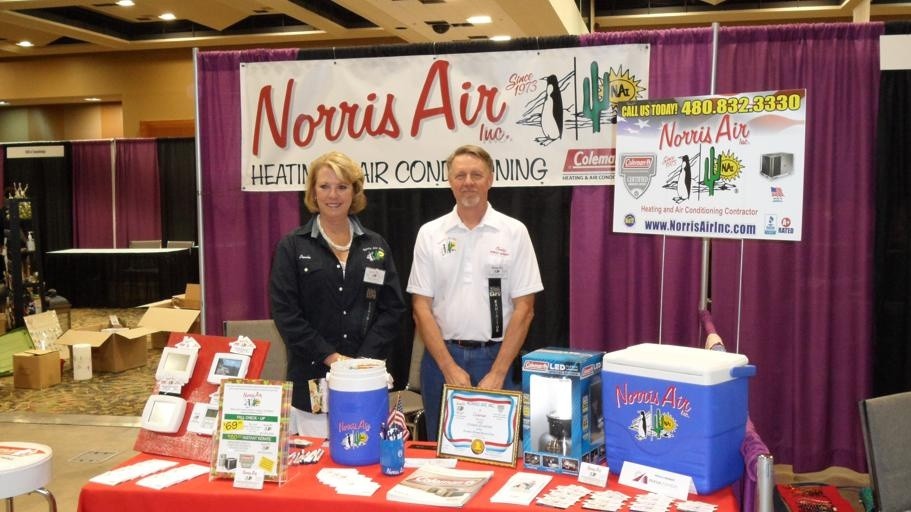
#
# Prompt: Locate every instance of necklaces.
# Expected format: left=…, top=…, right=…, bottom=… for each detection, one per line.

left=315, top=213, right=354, bottom=252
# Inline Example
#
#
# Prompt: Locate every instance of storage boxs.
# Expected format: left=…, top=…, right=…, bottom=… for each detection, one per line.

left=13, top=350, right=62, bottom=391
left=55, top=323, right=159, bottom=373
left=598, top=342, right=756, bottom=496
left=133, top=283, right=204, bottom=349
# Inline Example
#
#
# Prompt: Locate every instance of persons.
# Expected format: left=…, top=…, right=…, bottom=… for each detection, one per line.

left=405, top=143, right=545, bottom=441
left=268, top=151, right=408, bottom=439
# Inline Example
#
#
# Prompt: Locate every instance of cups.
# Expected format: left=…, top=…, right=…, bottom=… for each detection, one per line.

left=379, top=428, right=404, bottom=476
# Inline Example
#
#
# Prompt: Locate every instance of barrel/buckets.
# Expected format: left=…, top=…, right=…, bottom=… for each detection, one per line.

left=326, top=357, right=389, bottom=465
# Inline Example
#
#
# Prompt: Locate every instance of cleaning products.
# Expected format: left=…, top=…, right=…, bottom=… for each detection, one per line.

left=26, top=231, right=36, bottom=251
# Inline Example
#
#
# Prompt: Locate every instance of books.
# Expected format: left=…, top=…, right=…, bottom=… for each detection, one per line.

left=386, top=464, right=495, bottom=508
left=775, top=483, right=855, bottom=512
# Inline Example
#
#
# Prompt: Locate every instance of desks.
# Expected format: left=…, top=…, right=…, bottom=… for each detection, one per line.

left=45, top=247, right=189, bottom=308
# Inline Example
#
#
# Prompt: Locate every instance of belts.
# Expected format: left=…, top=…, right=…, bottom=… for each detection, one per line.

left=452, top=339, right=498, bottom=349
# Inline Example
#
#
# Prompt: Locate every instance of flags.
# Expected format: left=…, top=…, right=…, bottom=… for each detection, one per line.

left=387, top=394, right=411, bottom=444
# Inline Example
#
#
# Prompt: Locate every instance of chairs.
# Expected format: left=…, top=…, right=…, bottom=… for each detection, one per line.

left=223, top=317, right=288, bottom=383
left=128, top=240, right=162, bottom=248
left=386, top=324, right=429, bottom=442
left=167, top=240, right=194, bottom=254
left=857, top=391, right=911, bottom=511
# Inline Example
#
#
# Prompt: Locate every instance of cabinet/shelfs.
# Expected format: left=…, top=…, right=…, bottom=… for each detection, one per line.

left=1, top=198, right=45, bottom=332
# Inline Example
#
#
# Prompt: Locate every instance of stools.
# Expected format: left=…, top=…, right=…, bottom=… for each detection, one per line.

left=78, top=440, right=736, bottom=512
left=45, top=296, right=73, bottom=370
left=0, top=441, right=58, bottom=511
left=45, top=295, right=72, bottom=329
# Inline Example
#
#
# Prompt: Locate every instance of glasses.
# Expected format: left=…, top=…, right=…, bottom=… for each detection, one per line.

left=451, top=171, right=487, bottom=181
